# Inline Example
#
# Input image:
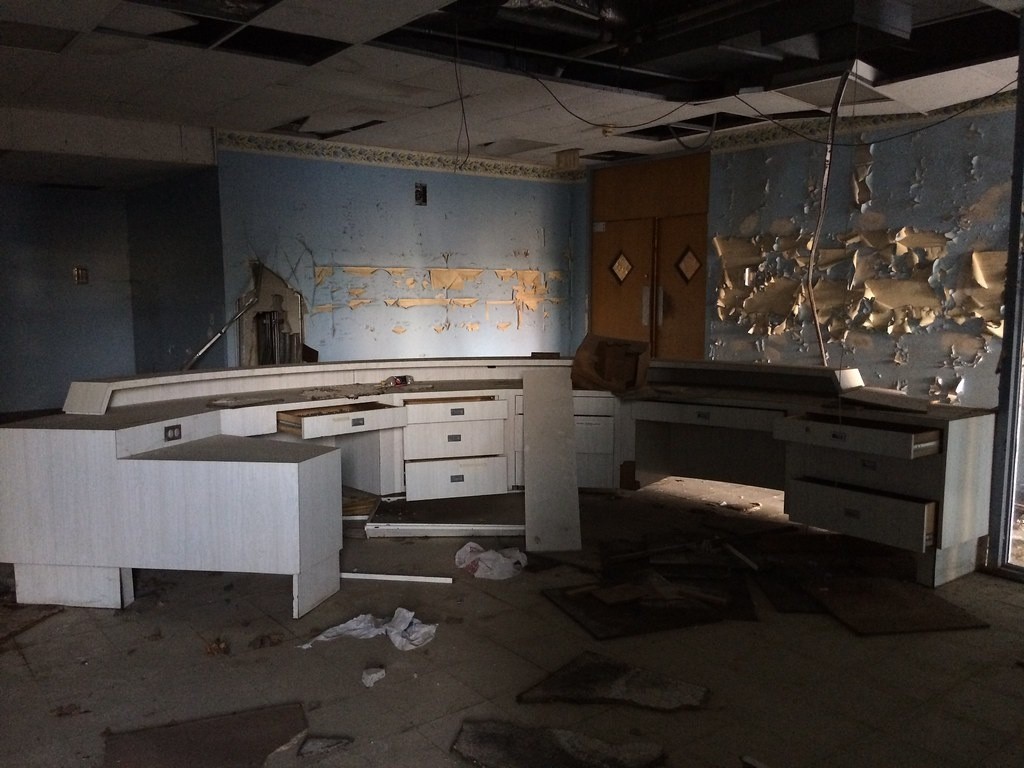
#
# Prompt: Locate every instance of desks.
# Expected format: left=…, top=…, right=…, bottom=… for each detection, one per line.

left=0, top=355, right=996, bottom=619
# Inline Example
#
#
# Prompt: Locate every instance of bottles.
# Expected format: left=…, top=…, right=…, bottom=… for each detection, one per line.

left=382, top=375, right=414, bottom=388
